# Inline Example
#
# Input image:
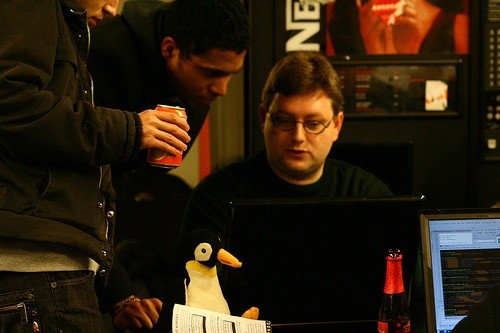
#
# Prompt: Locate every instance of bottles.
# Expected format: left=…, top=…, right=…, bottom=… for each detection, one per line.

left=378, top=249, right=411, bottom=333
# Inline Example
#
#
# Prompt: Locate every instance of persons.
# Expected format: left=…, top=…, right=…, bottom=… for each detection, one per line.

left=113, top=52, right=428, bottom=333
left=86, top=0, right=250, bottom=333
left=0, top=0, right=192, bottom=333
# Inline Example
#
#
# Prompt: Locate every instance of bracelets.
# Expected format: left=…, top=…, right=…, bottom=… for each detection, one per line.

left=112, top=295, right=135, bottom=317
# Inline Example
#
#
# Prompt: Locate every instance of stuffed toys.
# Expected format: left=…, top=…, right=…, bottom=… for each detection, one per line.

left=148, top=227, right=260, bottom=333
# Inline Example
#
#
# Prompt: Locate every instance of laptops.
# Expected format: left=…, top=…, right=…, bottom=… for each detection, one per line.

left=222, top=197, right=419, bottom=333
left=419, top=209, right=500, bottom=333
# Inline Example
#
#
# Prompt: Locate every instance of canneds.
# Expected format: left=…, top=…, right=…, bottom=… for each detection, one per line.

left=147, top=105, right=186, bottom=170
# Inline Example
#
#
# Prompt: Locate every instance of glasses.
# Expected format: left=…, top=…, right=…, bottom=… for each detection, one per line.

left=266, top=110, right=335, bottom=134
